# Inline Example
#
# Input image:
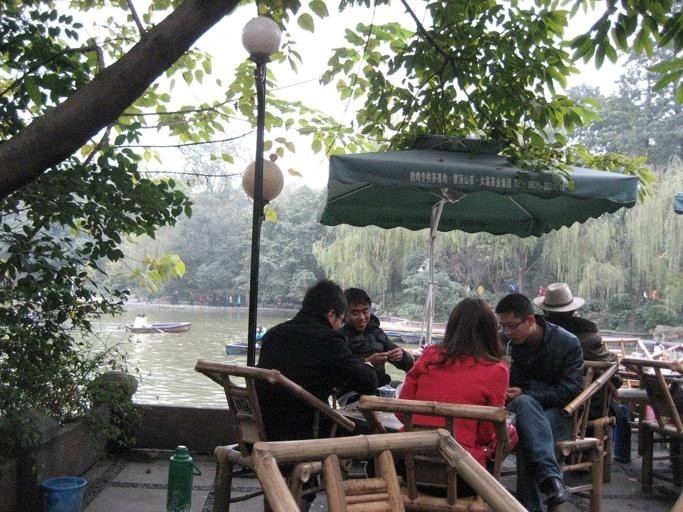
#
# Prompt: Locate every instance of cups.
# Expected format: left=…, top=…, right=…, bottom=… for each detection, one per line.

left=630, top=379, right=640, bottom=388
left=376, top=384, right=396, bottom=399
left=622, top=379, right=631, bottom=388
left=631, top=351, right=641, bottom=361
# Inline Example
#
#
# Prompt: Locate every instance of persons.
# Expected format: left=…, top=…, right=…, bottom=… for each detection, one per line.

left=133, top=313, right=143, bottom=328
left=142, top=315, right=150, bottom=329
left=253, top=280, right=415, bottom=441
left=255, top=327, right=259, bottom=342
left=532, top=282, right=630, bottom=463
left=393, top=296, right=519, bottom=497
left=494, top=292, right=584, bottom=510
left=259, top=326, right=266, bottom=341
left=642, top=285, right=662, bottom=303
left=140, top=287, right=245, bottom=307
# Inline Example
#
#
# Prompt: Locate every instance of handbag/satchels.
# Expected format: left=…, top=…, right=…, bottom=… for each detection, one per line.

left=477, top=420, right=519, bottom=460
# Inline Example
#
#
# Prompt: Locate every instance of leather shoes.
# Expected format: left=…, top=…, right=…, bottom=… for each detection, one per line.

left=540, top=477, right=568, bottom=507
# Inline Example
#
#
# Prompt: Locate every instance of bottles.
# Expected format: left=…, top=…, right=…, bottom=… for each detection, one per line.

left=653, top=341, right=676, bottom=361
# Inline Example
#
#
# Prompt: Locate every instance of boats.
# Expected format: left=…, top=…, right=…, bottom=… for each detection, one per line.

left=124, top=322, right=191, bottom=333
left=385, top=331, right=400, bottom=342
left=225, top=341, right=261, bottom=357
left=399, top=332, right=426, bottom=344
left=26, top=312, right=47, bottom=323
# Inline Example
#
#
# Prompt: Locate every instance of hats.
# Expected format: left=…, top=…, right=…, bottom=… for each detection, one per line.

left=533, top=282, right=586, bottom=313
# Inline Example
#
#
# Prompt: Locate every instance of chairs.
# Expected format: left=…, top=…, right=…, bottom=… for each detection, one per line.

left=620, top=358, right=682, bottom=496
left=358, top=395, right=511, bottom=511
left=250, top=431, right=527, bottom=512
left=195, top=362, right=355, bottom=512
left=549, top=361, right=618, bottom=512
left=600, top=335, right=658, bottom=455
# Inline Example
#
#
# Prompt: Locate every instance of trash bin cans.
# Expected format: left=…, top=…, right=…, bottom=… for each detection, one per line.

left=41, top=477, right=88, bottom=512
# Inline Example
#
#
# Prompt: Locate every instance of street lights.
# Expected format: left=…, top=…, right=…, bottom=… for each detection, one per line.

left=241, top=15, right=284, bottom=370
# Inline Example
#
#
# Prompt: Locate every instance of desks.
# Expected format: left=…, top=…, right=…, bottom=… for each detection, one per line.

left=340, top=402, right=414, bottom=432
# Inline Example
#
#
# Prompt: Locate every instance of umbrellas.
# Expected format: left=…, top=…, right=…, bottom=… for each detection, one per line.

left=313, top=111, right=638, bottom=356
left=674, top=193, right=683, bottom=215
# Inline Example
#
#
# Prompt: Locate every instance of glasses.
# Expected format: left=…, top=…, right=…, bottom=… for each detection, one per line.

left=497, top=319, right=527, bottom=334
